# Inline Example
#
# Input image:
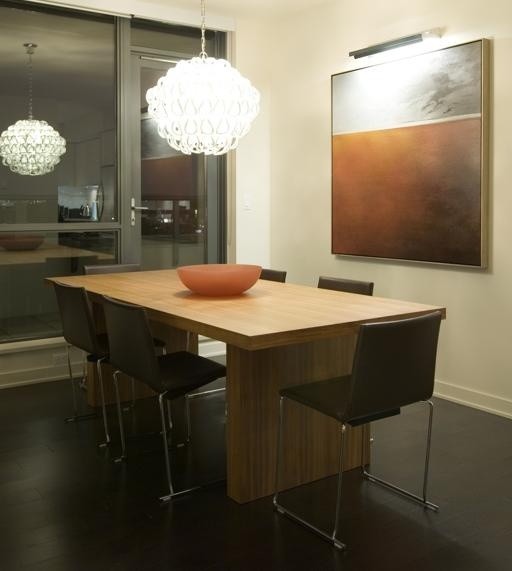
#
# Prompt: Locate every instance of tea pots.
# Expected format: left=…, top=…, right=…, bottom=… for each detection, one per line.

left=79, top=204, right=90, bottom=217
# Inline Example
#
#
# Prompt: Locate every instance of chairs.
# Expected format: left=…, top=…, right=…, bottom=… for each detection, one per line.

left=317, top=276, right=374, bottom=296
left=273, top=309, right=442, bottom=553
left=259, top=268, right=287, bottom=283
left=53, top=281, right=227, bottom=504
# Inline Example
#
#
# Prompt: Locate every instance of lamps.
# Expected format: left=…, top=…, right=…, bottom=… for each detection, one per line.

left=145, top=1, right=261, bottom=157
left=0, top=42, right=67, bottom=177
left=348, top=27, right=441, bottom=60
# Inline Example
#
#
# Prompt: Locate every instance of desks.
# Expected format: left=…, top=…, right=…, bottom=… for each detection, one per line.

left=42, top=268, right=445, bottom=506
left=0, top=243, right=101, bottom=268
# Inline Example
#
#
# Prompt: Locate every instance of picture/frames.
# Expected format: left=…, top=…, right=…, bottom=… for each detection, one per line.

left=330, top=38, right=490, bottom=269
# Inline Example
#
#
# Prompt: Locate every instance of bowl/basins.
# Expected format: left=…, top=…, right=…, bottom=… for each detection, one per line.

left=177, top=263, right=263, bottom=296
left=0, top=234, right=45, bottom=251
